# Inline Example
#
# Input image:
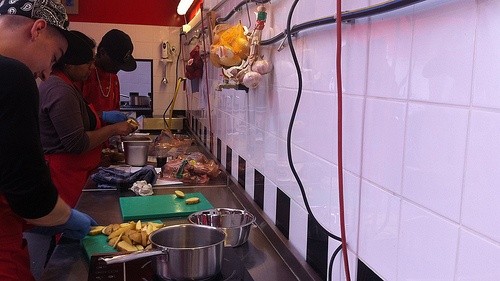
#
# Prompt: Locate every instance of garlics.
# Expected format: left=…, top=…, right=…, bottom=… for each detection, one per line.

left=243, top=56, right=272, bottom=88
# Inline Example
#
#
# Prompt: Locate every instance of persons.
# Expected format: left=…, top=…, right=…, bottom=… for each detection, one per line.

left=24, top=31, right=139, bottom=281
left=86, top=28, right=137, bottom=146
left=0, top=0, right=98, bottom=281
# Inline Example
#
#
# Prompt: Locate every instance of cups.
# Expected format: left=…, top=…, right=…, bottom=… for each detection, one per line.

left=157, top=152, right=166, bottom=168
left=114, top=165, right=131, bottom=197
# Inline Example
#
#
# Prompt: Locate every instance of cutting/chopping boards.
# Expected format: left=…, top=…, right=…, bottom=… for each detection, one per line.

left=119, top=192, right=215, bottom=221
left=80, top=220, right=166, bottom=261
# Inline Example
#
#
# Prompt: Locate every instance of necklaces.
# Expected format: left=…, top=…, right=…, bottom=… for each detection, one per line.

left=94, top=67, right=111, bottom=97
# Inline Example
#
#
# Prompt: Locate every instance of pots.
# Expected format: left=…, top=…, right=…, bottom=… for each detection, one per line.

left=148, top=223, right=227, bottom=281
left=120, top=94, right=150, bottom=106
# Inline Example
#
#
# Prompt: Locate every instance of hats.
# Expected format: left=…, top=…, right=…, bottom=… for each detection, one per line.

left=102, top=29, right=137, bottom=71
left=0, top=0, right=92, bottom=64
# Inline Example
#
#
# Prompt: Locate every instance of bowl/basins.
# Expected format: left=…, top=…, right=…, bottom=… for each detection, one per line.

left=188, top=208, right=257, bottom=247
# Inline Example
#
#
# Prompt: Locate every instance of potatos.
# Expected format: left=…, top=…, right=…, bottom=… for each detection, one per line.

left=91, top=220, right=164, bottom=254
left=185, top=197, right=200, bottom=204
left=174, top=190, right=184, bottom=197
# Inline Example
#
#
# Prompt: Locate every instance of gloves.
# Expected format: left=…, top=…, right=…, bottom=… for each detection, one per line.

left=55, top=208, right=98, bottom=239
left=103, top=111, right=128, bottom=124
